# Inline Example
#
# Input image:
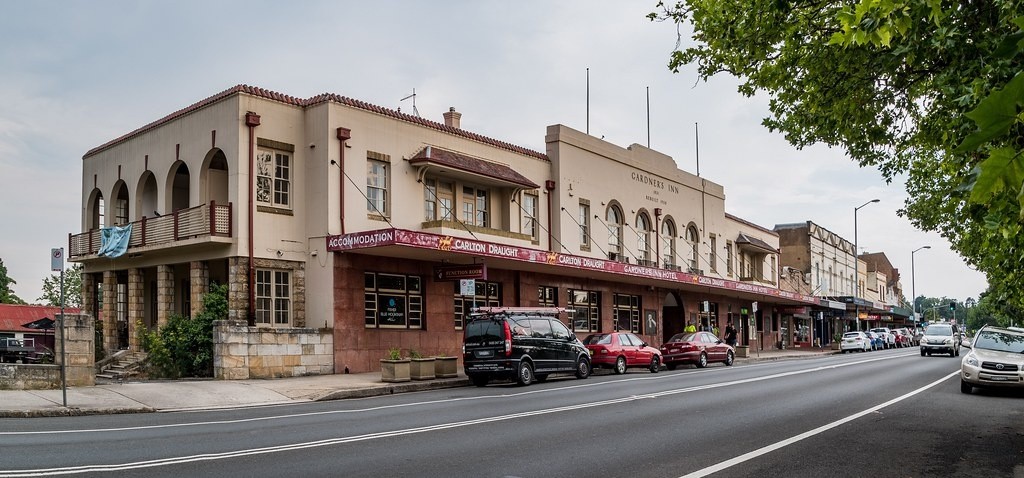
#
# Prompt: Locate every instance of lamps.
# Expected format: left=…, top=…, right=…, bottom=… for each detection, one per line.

left=277, top=250, right=283, bottom=257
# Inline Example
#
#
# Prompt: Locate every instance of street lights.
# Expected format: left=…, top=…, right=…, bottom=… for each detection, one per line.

left=855, top=198, right=880, bottom=331
left=912, top=245, right=931, bottom=346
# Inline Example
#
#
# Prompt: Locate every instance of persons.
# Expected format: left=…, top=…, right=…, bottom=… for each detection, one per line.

left=117, top=316, right=130, bottom=350
left=684, top=320, right=739, bottom=356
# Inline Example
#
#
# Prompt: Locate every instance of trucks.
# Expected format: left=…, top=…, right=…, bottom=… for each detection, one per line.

left=0, top=337, right=35, bottom=364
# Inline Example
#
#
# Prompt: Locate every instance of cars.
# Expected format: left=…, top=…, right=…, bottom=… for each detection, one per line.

left=840, top=327, right=924, bottom=353
left=581, top=332, right=662, bottom=374
left=660, top=332, right=736, bottom=370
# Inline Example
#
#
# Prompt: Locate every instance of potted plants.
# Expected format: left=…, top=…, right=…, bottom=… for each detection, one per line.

left=380, top=346, right=411, bottom=382
left=831, top=333, right=841, bottom=350
left=404, top=349, right=436, bottom=380
left=429, top=352, right=458, bottom=378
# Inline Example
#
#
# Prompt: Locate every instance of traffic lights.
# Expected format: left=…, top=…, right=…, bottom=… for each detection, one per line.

left=950, top=302, right=955, bottom=310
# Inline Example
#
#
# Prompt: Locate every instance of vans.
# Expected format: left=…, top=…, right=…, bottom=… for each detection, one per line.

left=462, top=314, right=591, bottom=386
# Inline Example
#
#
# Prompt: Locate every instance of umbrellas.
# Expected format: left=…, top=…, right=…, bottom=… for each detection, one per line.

left=20, top=317, right=55, bottom=357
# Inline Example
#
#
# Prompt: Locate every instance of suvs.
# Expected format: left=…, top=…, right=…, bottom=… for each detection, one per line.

left=961, top=324, right=1024, bottom=394
left=920, top=323, right=962, bottom=357
